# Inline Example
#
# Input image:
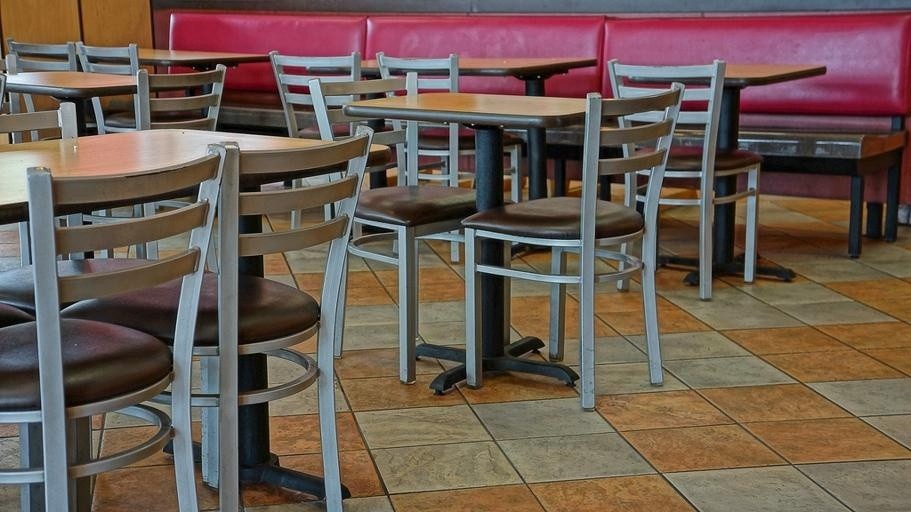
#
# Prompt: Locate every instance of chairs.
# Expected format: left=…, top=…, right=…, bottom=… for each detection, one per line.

left=0, top=4, right=909, bottom=411
left=62, top=123, right=375, bottom=511
left=1, top=144, right=227, bottom=511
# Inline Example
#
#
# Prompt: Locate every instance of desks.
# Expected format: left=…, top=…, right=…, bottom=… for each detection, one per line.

left=0, top=129, right=396, bottom=504
